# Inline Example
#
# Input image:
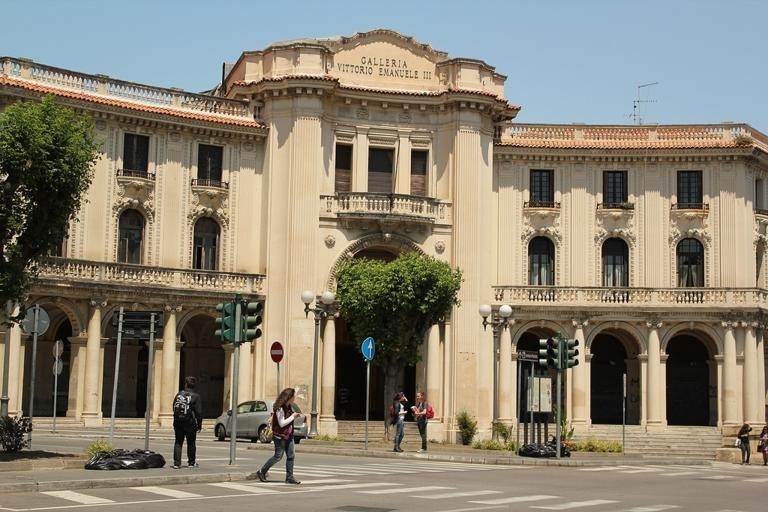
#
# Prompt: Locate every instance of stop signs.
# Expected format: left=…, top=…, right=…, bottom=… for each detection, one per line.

left=269, top=341, right=283, bottom=363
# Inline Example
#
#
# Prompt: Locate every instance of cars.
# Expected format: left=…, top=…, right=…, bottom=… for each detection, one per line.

left=213, top=398, right=309, bottom=444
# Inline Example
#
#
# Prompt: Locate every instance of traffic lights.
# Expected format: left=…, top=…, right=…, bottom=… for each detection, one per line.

left=563, top=337, right=580, bottom=369
left=238, top=296, right=264, bottom=344
left=547, top=337, right=562, bottom=369
left=214, top=301, right=234, bottom=344
left=538, top=336, right=552, bottom=370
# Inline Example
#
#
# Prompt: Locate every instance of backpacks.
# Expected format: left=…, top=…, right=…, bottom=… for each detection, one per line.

left=417, top=400, right=434, bottom=419
left=389, top=404, right=395, bottom=417
left=173, top=390, right=194, bottom=423
left=262, top=406, right=282, bottom=443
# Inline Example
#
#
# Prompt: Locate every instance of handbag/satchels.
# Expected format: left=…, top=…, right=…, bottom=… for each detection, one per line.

left=734, top=438, right=742, bottom=447
left=757, top=445, right=762, bottom=452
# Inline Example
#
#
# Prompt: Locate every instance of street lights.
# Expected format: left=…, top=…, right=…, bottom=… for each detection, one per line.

left=300, top=287, right=344, bottom=435
left=479, top=303, right=519, bottom=446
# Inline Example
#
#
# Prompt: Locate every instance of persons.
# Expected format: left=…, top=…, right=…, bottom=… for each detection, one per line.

left=759, top=427, right=768, bottom=465
left=738, top=424, right=752, bottom=465
left=169, top=376, right=202, bottom=469
left=256, top=388, right=301, bottom=484
left=389, top=391, right=408, bottom=452
left=412, top=391, right=429, bottom=453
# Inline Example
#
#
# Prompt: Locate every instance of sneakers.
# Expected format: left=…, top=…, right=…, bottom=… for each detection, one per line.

left=417, top=448, right=428, bottom=452
left=393, top=448, right=404, bottom=452
left=256, top=469, right=267, bottom=482
left=188, top=462, right=199, bottom=468
left=174, top=464, right=182, bottom=469
left=285, top=478, right=301, bottom=484
